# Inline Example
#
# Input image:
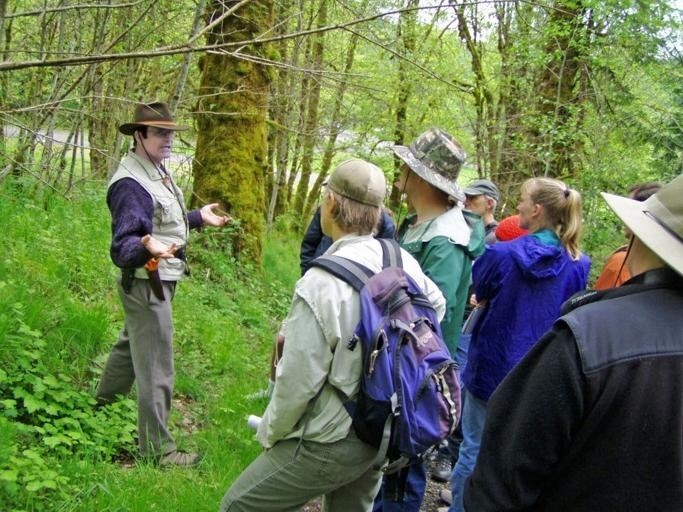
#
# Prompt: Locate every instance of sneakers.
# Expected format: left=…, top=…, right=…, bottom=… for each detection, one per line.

left=431, top=455, right=452, bottom=483
left=147, top=450, right=203, bottom=469
left=439, top=488, right=452, bottom=505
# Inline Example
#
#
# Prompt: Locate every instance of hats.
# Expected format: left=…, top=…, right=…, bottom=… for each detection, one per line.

left=390, top=127, right=466, bottom=202
left=599, top=174, right=683, bottom=278
left=323, top=157, right=386, bottom=206
left=463, top=179, right=500, bottom=202
left=118, top=102, right=190, bottom=136
left=494, top=215, right=527, bottom=241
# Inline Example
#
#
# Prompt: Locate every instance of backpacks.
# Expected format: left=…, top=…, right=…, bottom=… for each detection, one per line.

left=312, top=237, right=462, bottom=474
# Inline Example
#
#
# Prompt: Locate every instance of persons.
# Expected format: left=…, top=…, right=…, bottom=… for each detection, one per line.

left=219, top=128, right=484, bottom=511
left=91, top=101, right=234, bottom=471
left=430, top=174, right=683, bottom=510
